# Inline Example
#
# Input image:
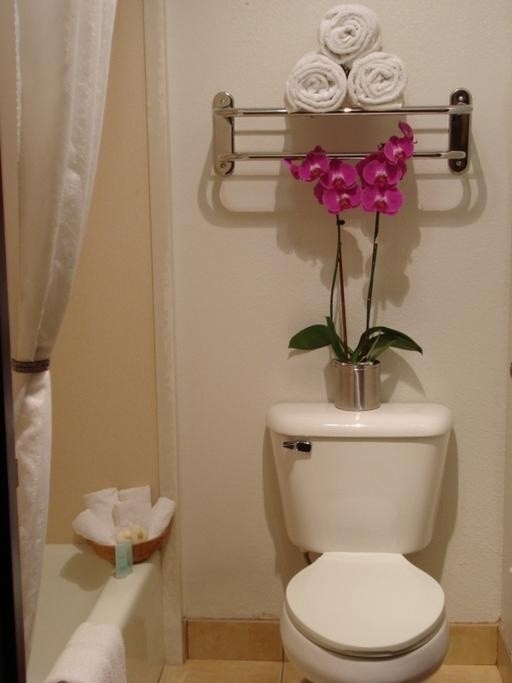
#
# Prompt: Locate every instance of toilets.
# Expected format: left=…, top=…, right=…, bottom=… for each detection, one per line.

left=264, top=400, right=453, bottom=683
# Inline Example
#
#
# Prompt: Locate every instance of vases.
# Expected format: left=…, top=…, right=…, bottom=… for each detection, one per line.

left=331, top=358, right=383, bottom=412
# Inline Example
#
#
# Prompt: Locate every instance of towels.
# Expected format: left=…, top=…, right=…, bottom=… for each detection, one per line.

left=285, top=54, right=347, bottom=115
left=110, top=501, right=148, bottom=541
left=83, top=487, right=116, bottom=524
left=346, top=51, right=407, bottom=111
left=145, top=497, right=176, bottom=539
left=319, top=4, right=380, bottom=62
left=120, top=485, right=151, bottom=508
left=70, top=509, right=117, bottom=549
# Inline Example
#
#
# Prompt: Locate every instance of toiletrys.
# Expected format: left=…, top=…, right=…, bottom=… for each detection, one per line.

left=115, top=537, right=132, bottom=579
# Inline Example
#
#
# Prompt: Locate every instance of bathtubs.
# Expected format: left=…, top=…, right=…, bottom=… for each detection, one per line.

left=22, top=542, right=164, bottom=682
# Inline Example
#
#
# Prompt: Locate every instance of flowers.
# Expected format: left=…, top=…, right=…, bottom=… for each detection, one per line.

left=278, top=122, right=423, bottom=361
